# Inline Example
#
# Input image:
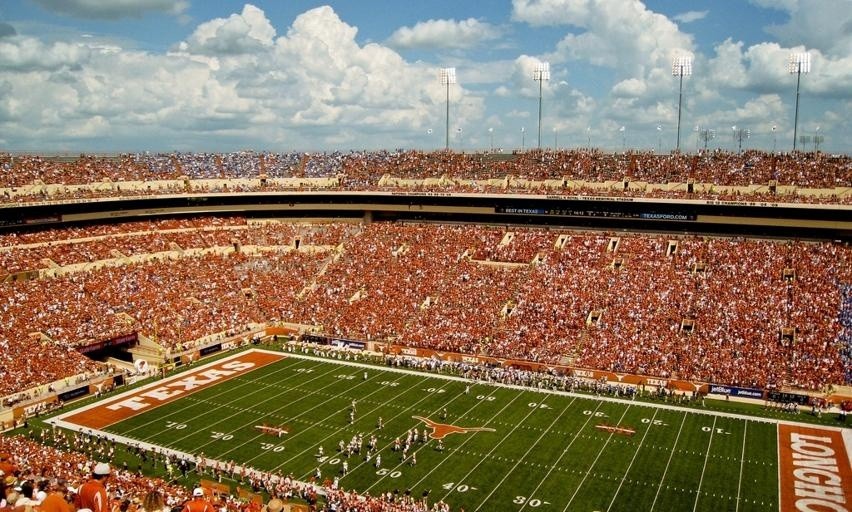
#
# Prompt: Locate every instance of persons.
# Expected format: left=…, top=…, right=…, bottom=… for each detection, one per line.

left=227, top=151, right=852, bottom=427
left=0, top=152, right=228, bottom=512
left=228, top=427, right=481, bottom=511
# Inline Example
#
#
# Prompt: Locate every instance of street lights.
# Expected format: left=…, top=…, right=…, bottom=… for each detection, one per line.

left=770, top=126, right=777, bottom=152
left=440, top=66, right=456, bottom=149
left=656, top=127, right=663, bottom=148
left=427, top=128, right=434, bottom=152
left=815, top=127, right=821, bottom=151
left=488, top=128, right=495, bottom=151
left=672, top=54, right=691, bottom=150
left=731, top=126, right=737, bottom=141
left=619, top=127, right=626, bottom=147
left=694, top=127, right=699, bottom=149
left=553, top=127, right=560, bottom=152
left=788, top=51, right=812, bottom=149
left=520, top=127, right=526, bottom=150
left=457, top=127, right=465, bottom=155
left=530, top=62, right=551, bottom=147
left=584, top=127, right=591, bottom=147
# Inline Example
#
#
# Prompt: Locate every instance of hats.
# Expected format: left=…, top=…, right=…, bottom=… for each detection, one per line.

left=7, top=492, right=16, bottom=503
left=1, top=454, right=8, bottom=459
left=0, top=470, right=5, bottom=476
left=6, top=475, right=17, bottom=485
left=94, top=462, right=110, bottom=475
left=193, top=488, right=203, bottom=497
left=58, top=479, right=66, bottom=484
left=261, top=499, right=290, bottom=512
